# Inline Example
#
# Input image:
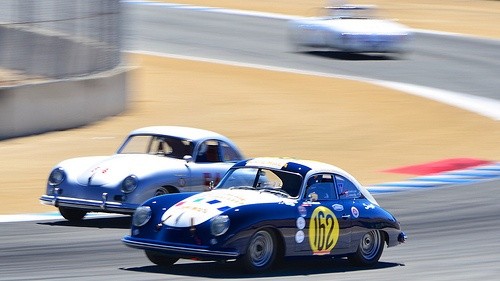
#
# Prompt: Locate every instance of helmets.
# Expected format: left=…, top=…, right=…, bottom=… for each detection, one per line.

left=188, top=141, right=208, bottom=156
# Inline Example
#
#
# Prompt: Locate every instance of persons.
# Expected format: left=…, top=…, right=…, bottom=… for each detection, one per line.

left=291, top=175, right=322, bottom=198
left=189, top=142, right=208, bottom=162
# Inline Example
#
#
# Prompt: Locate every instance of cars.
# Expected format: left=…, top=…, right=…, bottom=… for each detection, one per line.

left=39, top=125, right=270, bottom=222
left=285, top=3, right=414, bottom=56
left=121, top=157, right=408, bottom=276
